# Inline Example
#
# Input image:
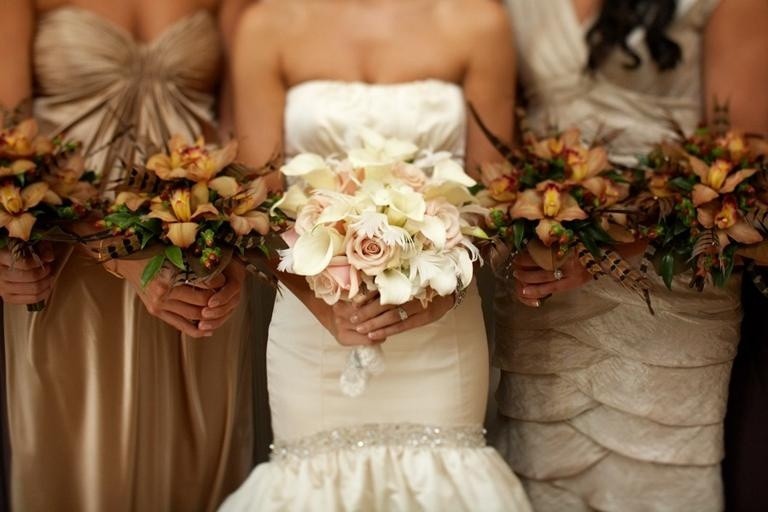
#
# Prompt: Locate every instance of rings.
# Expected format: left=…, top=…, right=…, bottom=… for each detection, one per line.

left=553, top=269, right=563, bottom=281
left=396, top=306, right=409, bottom=321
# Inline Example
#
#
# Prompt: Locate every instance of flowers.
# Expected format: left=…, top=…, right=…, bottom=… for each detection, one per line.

left=1, top=102, right=99, bottom=258
left=270, top=129, right=490, bottom=309
left=466, top=101, right=658, bottom=300
left=631, top=130, right=767, bottom=296
left=99, top=132, right=292, bottom=289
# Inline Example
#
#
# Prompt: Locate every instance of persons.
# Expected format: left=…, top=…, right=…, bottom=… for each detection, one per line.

left=214, top=2, right=538, bottom=509
left=485, top=0, right=768, bottom=510
left=1, top=0, right=268, bottom=509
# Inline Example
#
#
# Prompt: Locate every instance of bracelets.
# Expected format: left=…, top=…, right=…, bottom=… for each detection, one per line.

left=96, top=236, right=125, bottom=281
left=500, top=241, right=524, bottom=280
left=451, top=278, right=469, bottom=312
